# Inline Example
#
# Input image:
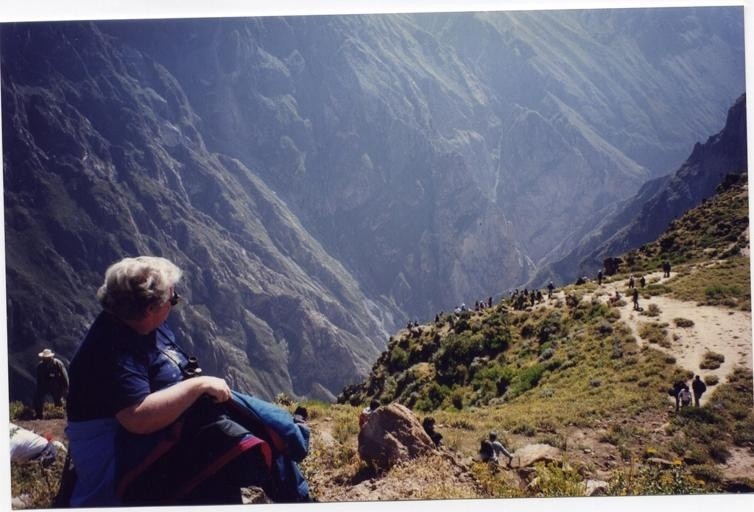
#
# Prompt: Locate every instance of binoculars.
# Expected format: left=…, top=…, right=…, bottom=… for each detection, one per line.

left=182, top=356, right=202, bottom=377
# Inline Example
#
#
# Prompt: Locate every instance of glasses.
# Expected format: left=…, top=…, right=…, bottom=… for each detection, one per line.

left=167, top=290, right=177, bottom=307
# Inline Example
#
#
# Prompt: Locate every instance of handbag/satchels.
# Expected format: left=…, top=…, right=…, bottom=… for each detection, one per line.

left=231, top=388, right=310, bottom=464
left=19, top=442, right=57, bottom=476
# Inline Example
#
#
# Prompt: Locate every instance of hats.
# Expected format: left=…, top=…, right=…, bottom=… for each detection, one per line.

left=38, top=349, right=54, bottom=359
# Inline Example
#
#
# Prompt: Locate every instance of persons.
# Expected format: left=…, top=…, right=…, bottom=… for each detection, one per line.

left=33, top=348, right=70, bottom=419
left=662, top=262, right=668, bottom=277
left=692, top=375, right=706, bottom=407
left=678, top=386, right=692, bottom=405
left=597, top=269, right=605, bottom=284
left=11, top=422, right=66, bottom=477
left=628, top=275, right=635, bottom=288
left=405, top=279, right=556, bottom=331
left=673, top=380, right=687, bottom=412
left=360, top=399, right=380, bottom=431
left=54, top=256, right=320, bottom=503
left=640, top=275, right=646, bottom=288
left=486, top=431, right=516, bottom=468
left=666, top=262, right=671, bottom=277
left=633, top=289, right=640, bottom=309
left=422, top=416, right=442, bottom=448
left=295, top=405, right=307, bottom=427
